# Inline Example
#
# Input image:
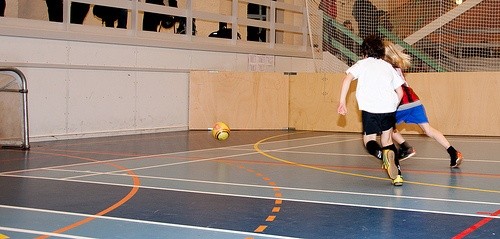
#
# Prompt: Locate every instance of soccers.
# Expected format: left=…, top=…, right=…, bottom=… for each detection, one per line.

left=212, top=122, right=231, bottom=141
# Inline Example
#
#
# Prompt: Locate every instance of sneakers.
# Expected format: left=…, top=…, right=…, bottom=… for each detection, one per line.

left=391, top=175, right=404, bottom=186
left=450, top=151, right=462, bottom=167
left=398, top=146, right=417, bottom=161
left=382, top=149, right=398, bottom=180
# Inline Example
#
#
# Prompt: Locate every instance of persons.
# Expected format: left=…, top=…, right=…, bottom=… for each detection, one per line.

left=384, top=42, right=462, bottom=168
left=337, top=37, right=404, bottom=185
left=340, top=20, right=354, bottom=67
left=46, top=0, right=196, bottom=36
left=352, top=0, right=379, bottom=39
